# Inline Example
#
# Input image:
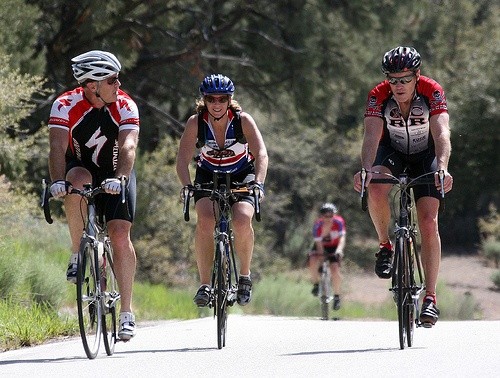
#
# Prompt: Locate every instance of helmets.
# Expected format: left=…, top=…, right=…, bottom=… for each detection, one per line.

left=381, top=46, right=423, bottom=76
left=199, top=73, right=235, bottom=95
left=320, top=203, right=337, bottom=214
left=71, top=50, right=121, bottom=83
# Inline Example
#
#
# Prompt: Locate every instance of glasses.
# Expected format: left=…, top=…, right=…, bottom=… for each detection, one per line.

left=386, top=71, right=417, bottom=84
left=322, top=215, right=334, bottom=219
left=203, top=95, right=230, bottom=103
left=95, top=77, right=119, bottom=86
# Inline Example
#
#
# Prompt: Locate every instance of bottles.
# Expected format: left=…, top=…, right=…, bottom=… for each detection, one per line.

left=409, top=236, right=414, bottom=275
left=97, top=242, right=107, bottom=291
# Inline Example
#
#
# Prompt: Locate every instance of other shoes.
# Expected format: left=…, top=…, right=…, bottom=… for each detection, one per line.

left=312, top=288, right=319, bottom=296
left=334, top=299, right=340, bottom=310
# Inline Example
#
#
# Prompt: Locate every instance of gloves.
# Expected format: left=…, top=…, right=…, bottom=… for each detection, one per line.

left=178, top=183, right=195, bottom=205
left=248, top=181, right=265, bottom=204
left=49, top=180, right=72, bottom=201
left=101, top=178, right=121, bottom=194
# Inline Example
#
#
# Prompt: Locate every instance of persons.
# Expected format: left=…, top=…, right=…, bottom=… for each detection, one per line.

left=309, top=203, right=346, bottom=310
left=175, top=74, right=269, bottom=307
left=48, top=50, right=139, bottom=338
left=353, top=47, right=453, bottom=325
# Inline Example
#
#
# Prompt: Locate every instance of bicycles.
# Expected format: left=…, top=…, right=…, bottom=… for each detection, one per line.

left=41, top=177, right=130, bottom=359
left=310, top=253, right=341, bottom=321
left=181, top=176, right=272, bottom=345
left=362, top=167, right=445, bottom=347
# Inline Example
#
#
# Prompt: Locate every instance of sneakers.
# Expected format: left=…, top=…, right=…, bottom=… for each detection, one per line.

left=237, top=270, right=252, bottom=307
left=193, top=285, right=211, bottom=306
left=375, top=248, right=395, bottom=278
left=66, top=253, right=81, bottom=282
left=118, top=311, right=135, bottom=339
left=420, top=296, right=440, bottom=324
